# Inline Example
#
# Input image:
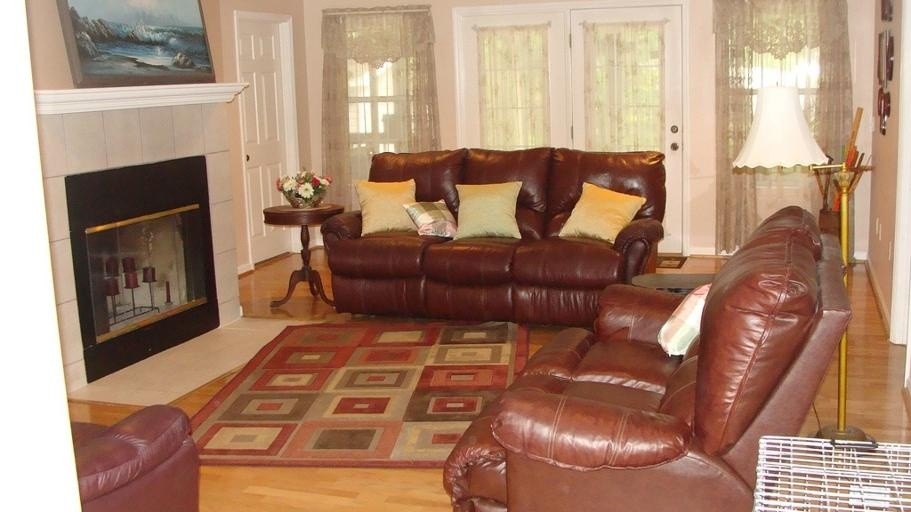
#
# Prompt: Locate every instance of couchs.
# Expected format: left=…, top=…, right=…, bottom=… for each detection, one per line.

left=442, top=204, right=854, bottom=512
left=320, top=146, right=666, bottom=329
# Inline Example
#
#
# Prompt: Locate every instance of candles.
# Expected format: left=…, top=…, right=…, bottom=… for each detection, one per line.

left=122, top=256, right=136, bottom=272
left=142, top=266, right=155, bottom=283
left=104, top=278, right=119, bottom=296
left=125, top=272, right=138, bottom=288
left=105, top=256, right=118, bottom=275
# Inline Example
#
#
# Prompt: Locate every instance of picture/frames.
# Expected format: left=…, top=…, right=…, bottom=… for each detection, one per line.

left=56, top=0, right=216, bottom=89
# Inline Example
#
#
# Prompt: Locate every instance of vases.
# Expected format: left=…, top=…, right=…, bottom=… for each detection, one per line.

left=285, top=196, right=324, bottom=210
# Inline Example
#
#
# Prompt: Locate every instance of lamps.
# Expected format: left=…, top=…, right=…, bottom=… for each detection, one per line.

left=731, top=78, right=879, bottom=456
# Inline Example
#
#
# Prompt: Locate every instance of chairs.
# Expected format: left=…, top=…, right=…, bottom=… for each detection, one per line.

left=69, top=404, right=199, bottom=512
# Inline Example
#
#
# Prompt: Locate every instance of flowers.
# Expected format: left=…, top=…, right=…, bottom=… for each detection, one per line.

left=275, top=170, right=333, bottom=203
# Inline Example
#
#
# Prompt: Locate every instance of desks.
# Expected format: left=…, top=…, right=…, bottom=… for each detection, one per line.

left=263, top=203, right=345, bottom=308
left=631, top=272, right=720, bottom=296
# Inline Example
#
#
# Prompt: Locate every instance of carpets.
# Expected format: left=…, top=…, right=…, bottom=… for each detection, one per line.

left=655, top=255, right=688, bottom=269
left=186, top=315, right=530, bottom=468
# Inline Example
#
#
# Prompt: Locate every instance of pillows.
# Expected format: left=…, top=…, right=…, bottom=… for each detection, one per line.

left=353, top=178, right=416, bottom=237
left=558, top=181, right=647, bottom=245
left=402, top=199, right=458, bottom=239
left=453, top=180, right=524, bottom=241
left=656, top=283, right=713, bottom=357
